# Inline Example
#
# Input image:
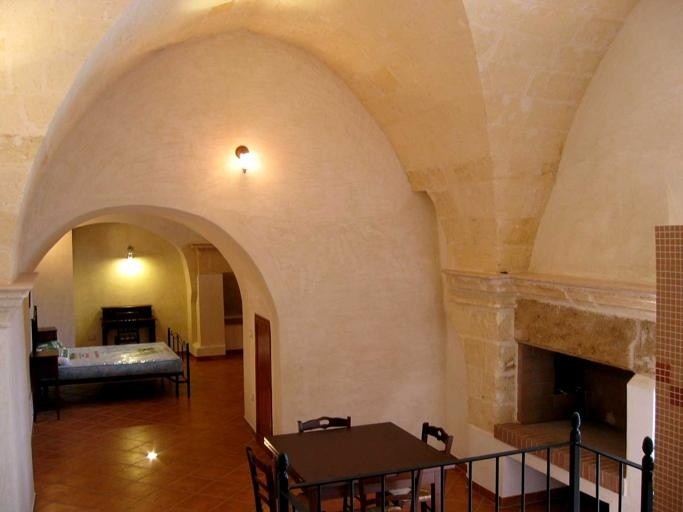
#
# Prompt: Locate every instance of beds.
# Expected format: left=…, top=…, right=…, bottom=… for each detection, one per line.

left=31, top=304, right=192, bottom=401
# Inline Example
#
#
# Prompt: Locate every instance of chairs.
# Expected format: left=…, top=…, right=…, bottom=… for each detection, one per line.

left=244, top=413, right=454, bottom=512
left=113, top=311, right=140, bottom=344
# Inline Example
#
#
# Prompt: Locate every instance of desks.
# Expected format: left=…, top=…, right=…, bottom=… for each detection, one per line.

left=29, top=347, right=61, bottom=423
left=97, top=304, right=158, bottom=345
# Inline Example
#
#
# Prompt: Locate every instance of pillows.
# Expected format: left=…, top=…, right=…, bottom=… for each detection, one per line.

left=35, top=339, right=72, bottom=365
